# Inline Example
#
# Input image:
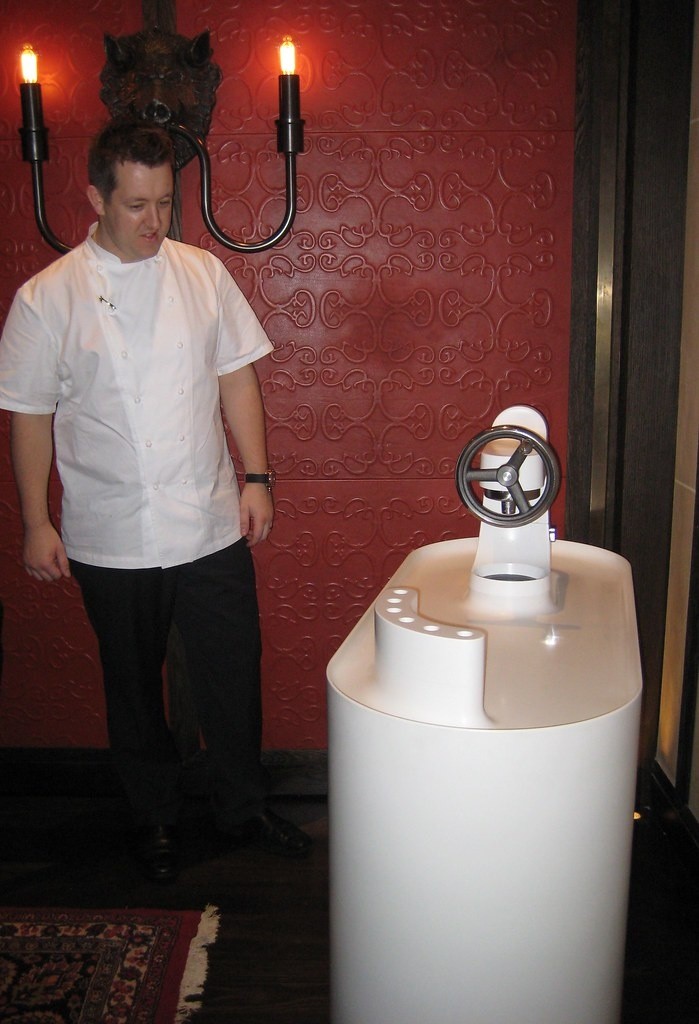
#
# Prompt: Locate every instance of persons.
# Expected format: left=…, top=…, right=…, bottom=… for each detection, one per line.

left=0, top=118, right=311, bottom=887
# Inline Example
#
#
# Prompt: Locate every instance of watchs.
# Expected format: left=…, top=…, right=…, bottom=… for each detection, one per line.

left=245, top=469, right=276, bottom=492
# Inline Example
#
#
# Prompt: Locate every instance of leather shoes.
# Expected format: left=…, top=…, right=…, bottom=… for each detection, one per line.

left=227, top=807, right=313, bottom=857
left=139, top=824, right=182, bottom=882
